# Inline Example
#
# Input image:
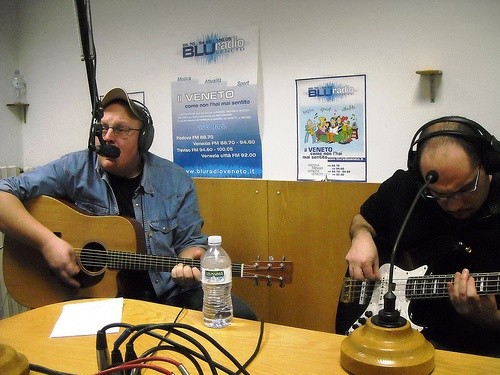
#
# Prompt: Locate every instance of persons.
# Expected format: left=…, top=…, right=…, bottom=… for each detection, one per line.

left=344, top=115, right=500, bottom=360
left=0, top=87, right=259, bottom=321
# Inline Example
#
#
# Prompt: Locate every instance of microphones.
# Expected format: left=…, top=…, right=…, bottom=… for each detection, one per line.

left=88, top=144, right=121, bottom=158
left=340, top=170, right=439, bottom=375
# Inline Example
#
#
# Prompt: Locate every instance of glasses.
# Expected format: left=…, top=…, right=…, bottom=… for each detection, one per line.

left=418, top=161, right=481, bottom=200
left=101, top=125, right=143, bottom=137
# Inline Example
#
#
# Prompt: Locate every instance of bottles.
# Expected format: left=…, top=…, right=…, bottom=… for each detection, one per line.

left=200, top=236, right=233, bottom=329
left=11, top=71, right=25, bottom=103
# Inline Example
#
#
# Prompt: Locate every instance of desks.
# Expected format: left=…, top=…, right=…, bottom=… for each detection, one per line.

left=0, top=298, right=500, bottom=375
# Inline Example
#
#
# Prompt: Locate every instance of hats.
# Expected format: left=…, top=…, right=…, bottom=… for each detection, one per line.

left=101, top=87, right=148, bottom=125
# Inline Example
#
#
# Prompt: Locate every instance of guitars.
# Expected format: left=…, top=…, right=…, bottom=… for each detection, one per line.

left=2, top=194, right=294, bottom=309
left=335, top=262, right=500, bottom=337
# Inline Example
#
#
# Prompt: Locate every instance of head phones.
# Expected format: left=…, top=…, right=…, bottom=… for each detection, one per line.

left=132, top=100, right=155, bottom=152
left=407, top=116, right=500, bottom=203
